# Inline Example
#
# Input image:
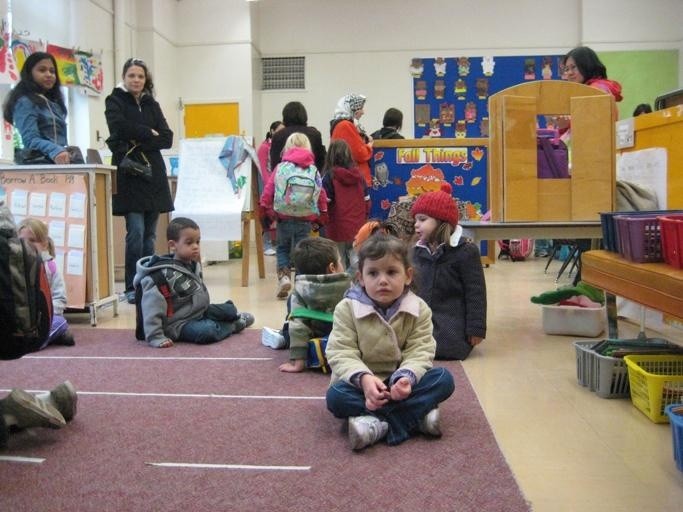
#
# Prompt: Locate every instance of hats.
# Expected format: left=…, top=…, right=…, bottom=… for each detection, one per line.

left=411, top=183, right=459, bottom=226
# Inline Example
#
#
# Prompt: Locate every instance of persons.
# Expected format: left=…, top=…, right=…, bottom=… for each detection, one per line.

left=261, top=182, right=485, bottom=373
left=15, top=216, right=76, bottom=350
left=632, top=101, right=654, bottom=117
left=104, top=56, right=174, bottom=304
left=0, top=204, right=49, bottom=361
left=2, top=49, right=84, bottom=165
left=554, top=46, right=622, bottom=141
left=324, top=220, right=456, bottom=452
left=1, top=378, right=78, bottom=454
left=256, top=93, right=405, bottom=296
left=131, top=215, right=255, bottom=349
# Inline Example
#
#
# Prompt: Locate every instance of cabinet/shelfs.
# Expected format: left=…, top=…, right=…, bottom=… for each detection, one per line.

left=112, top=174, right=178, bottom=284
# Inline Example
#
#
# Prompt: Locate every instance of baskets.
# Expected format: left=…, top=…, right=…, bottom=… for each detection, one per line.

left=597, top=210, right=683, bottom=272
left=623, top=354, right=682, bottom=424
left=664, top=404, right=683, bottom=470
left=571, top=337, right=680, bottom=399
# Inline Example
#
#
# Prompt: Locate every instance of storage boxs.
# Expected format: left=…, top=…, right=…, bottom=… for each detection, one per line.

left=540, top=299, right=607, bottom=339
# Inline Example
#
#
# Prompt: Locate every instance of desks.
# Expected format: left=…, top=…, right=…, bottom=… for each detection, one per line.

left=612, top=104, right=683, bottom=210
left=1, top=162, right=120, bottom=332
left=577, top=248, right=683, bottom=345
left=457, top=218, right=603, bottom=253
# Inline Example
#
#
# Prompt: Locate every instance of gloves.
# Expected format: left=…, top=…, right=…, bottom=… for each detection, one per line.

left=318, top=211, right=329, bottom=226
left=259, top=206, right=276, bottom=231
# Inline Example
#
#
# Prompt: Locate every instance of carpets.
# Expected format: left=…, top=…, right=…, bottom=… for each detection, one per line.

left=0, top=326, right=534, bottom=510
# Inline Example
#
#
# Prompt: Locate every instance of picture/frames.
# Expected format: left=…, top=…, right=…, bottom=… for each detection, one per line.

left=370, top=138, right=496, bottom=267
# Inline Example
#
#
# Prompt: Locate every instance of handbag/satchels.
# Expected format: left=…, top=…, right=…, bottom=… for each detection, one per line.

left=119, top=157, right=153, bottom=179
left=14, top=145, right=84, bottom=164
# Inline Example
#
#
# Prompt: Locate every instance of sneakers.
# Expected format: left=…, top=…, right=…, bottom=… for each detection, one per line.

left=2, top=389, right=68, bottom=433
left=234, top=312, right=254, bottom=330
left=264, top=249, right=276, bottom=255
left=348, top=415, right=388, bottom=450
left=276, top=276, right=292, bottom=298
left=262, top=327, right=286, bottom=350
left=423, top=408, right=443, bottom=437
left=49, top=380, right=77, bottom=421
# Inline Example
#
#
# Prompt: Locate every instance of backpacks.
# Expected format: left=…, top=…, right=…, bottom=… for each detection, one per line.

left=287, top=307, right=335, bottom=374
left=134, top=270, right=174, bottom=341
left=1, top=229, right=53, bottom=360
left=273, top=161, right=319, bottom=218
